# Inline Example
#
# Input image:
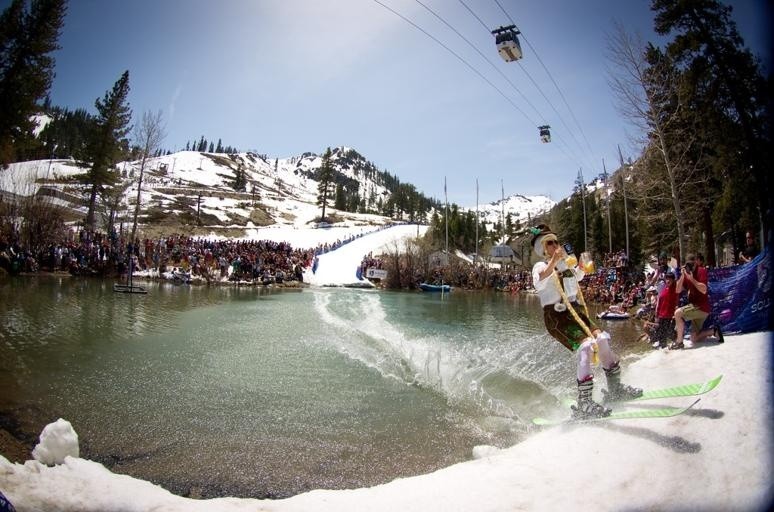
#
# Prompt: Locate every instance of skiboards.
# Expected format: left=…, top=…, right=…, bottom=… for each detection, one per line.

left=534, top=375, right=724, bottom=427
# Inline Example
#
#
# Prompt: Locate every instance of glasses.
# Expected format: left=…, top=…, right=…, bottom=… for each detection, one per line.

left=547, top=240, right=559, bottom=245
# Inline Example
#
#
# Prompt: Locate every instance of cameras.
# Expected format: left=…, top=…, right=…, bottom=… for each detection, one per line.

left=685, top=261, right=697, bottom=274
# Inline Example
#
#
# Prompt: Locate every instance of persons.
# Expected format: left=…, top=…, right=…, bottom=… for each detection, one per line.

left=528, top=225, right=642, bottom=419
left=579, top=232, right=761, bottom=350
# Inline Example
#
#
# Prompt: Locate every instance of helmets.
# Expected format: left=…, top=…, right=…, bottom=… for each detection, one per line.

left=533, top=234, right=556, bottom=257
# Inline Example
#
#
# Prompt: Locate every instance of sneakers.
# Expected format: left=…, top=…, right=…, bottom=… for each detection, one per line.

left=709, top=323, right=724, bottom=343
left=668, top=342, right=684, bottom=350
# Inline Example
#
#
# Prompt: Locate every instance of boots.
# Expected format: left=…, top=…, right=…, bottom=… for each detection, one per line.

left=602, top=360, right=643, bottom=394
left=576, top=374, right=612, bottom=413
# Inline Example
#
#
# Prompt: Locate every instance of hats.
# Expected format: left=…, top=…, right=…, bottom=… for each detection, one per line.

left=531, top=223, right=557, bottom=247
